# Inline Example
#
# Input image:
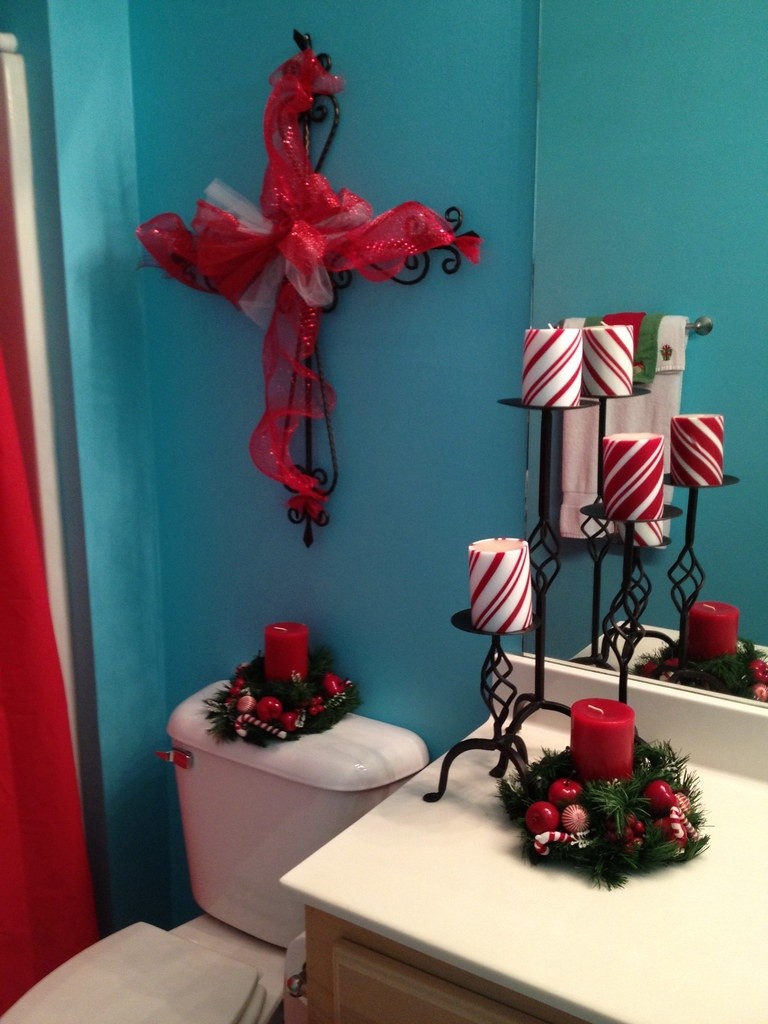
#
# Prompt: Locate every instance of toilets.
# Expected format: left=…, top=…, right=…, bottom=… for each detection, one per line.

left=0, top=679, right=431, bottom=1023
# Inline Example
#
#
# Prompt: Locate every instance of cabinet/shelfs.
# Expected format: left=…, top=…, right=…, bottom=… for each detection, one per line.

left=295, top=906, right=586, bottom=1023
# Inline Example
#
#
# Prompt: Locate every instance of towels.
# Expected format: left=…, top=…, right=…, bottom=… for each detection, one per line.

left=560, top=312, right=690, bottom=549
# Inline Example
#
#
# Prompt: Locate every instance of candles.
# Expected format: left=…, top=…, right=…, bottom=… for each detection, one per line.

left=671, top=414, right=724, bottom=486
left=571, top=699, right=636, bottom=784
left=522, top=326, right=583, bottom=407
left=264, top=622, right=307, bottom=682
left=614, top=520, right=662, bottom=547
left=582, top=324, right=633, bottom=398
left=687, top=602, right=738, bottom=659
left=468, top=537, right=532, bottom=632
left=602, top=434, right=663, bottom=519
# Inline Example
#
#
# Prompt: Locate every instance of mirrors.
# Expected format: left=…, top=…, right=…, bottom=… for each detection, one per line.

left=516, top=0, right=768, bottom=708
